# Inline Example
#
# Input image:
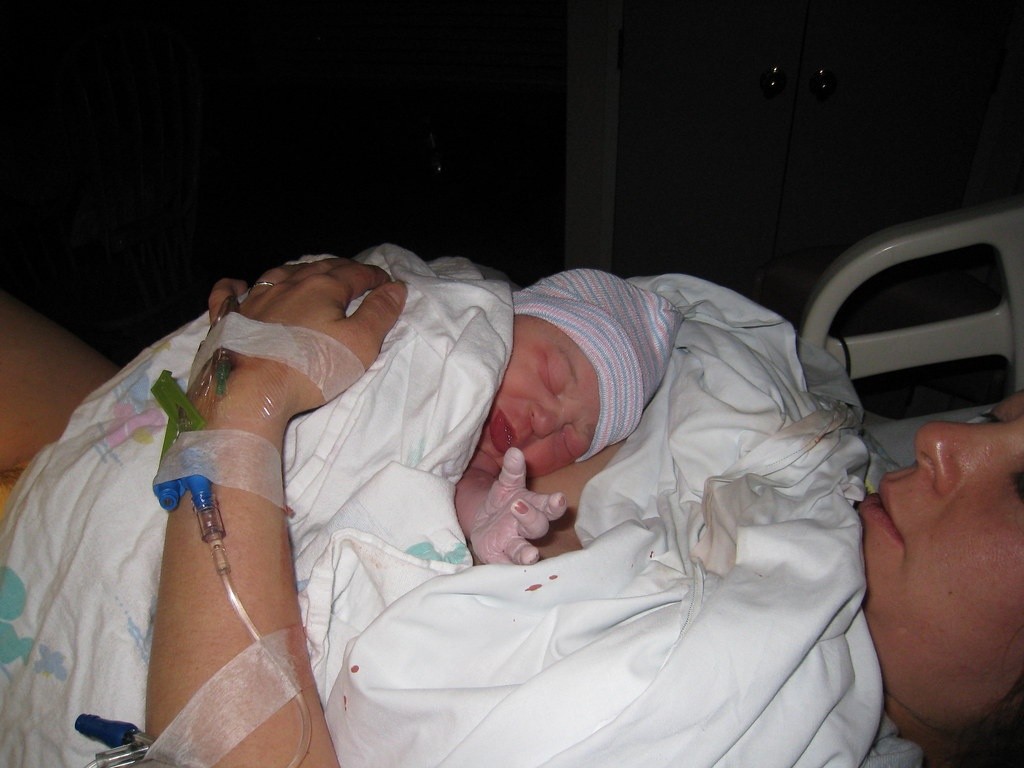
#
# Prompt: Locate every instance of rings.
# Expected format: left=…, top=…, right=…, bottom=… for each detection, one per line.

left=247, top=279, right=275, bottom=293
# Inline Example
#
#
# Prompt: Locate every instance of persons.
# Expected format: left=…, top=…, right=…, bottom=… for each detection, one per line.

left=0, top=242, right=1023, bottom=768
